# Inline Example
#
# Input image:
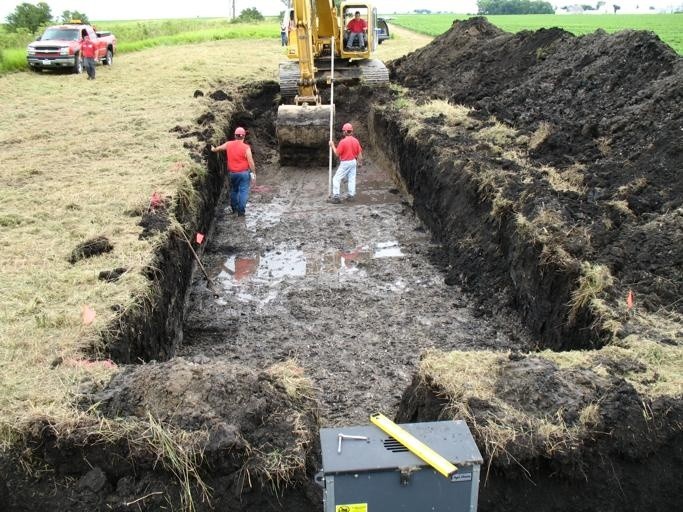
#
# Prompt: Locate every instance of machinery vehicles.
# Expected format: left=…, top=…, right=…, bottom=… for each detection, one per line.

left=276, top=1, right=390, bottom=165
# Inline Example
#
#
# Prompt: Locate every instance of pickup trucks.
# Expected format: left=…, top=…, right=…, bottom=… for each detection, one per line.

left=26, top=20, right=117, bottom=74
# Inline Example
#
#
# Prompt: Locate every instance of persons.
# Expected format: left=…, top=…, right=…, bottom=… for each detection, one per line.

left=280, top=23, right=287, bottom=46
left=345, top=11, right=368, bottom=50
left=210, top=127, right=256, bottom=217
left=329, top=123, right=363, bottom=204
left=78, top=34, right=98, bottom=80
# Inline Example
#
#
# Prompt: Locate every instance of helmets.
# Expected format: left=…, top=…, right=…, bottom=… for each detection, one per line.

left=235, top=127, right=245, bottom=135
left=341, top=123, right=353, bottom=131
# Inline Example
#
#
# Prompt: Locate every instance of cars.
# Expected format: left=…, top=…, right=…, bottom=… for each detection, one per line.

left=365, top=17, right=390, bottom=46
